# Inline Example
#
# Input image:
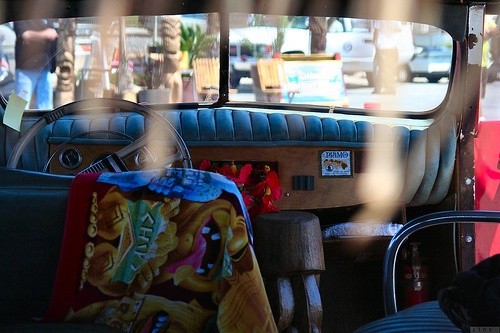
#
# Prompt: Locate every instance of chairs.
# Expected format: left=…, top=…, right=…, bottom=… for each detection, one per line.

left=0, top=168, right=279, bottom=333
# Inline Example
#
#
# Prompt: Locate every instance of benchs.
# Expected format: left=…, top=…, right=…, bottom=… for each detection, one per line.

left=194, top=57, right=238, bottom=102
left=257, top=57, right=300, bottom=104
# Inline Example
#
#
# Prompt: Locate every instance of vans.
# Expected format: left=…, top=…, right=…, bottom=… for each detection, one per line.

left=326, top=17, right=415, bottom=88
left=195, top=26, right=310, bottom=87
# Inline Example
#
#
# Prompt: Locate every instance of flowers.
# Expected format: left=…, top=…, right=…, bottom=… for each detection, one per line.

left=199, top=159, right=282, bottom=217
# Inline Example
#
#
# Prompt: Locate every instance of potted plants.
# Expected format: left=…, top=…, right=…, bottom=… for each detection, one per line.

left=128, top=40, right=170, bottom=104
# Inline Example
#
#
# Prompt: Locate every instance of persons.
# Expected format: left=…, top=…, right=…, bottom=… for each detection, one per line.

left=13, top=19, right=59, bottom=110
left=372, top=20, right=399, bottom=95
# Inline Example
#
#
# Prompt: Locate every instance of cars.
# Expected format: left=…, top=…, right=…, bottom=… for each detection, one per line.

left=75, top=23, right=150, bottom=66
left=397, top=32, right=454, bottom=83
